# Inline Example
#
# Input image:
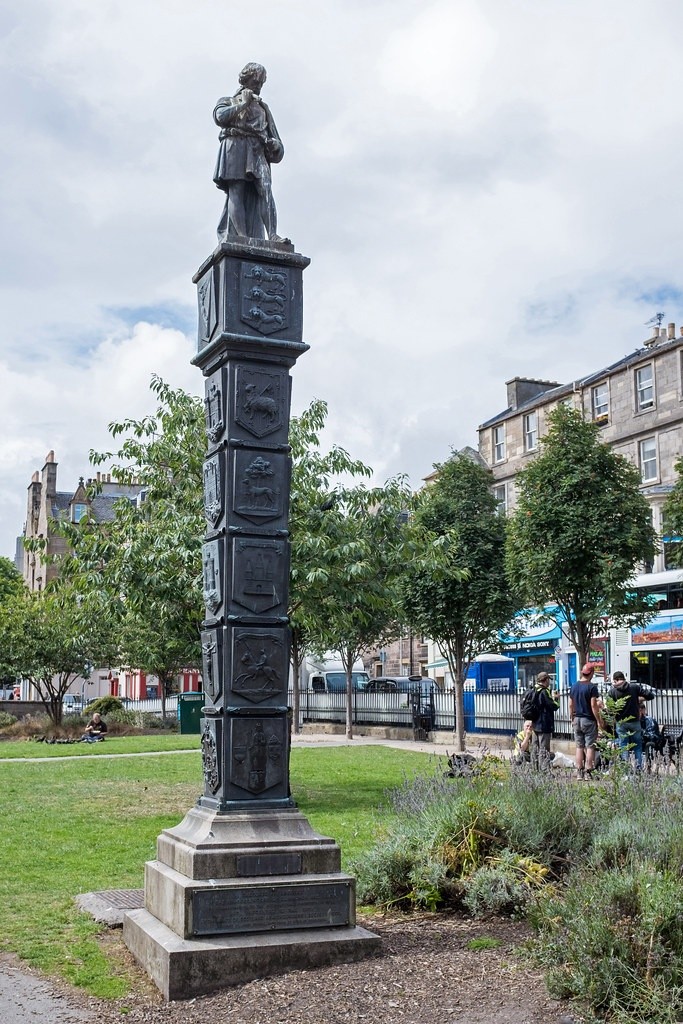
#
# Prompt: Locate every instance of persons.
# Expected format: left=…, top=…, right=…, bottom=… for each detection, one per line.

left=212, top=62, right=292, bottom=243
left=81, top=712, right=108, bottom=741
left=514, top=721, right=555, bottom=766
left=531, top=671, right=562, bottom=779
left=570, top=663, right=662, bottom=781
left=9, top=688, right=20, bottom=700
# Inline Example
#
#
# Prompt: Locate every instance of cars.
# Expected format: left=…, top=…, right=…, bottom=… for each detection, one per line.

left=87, top=696, right=131, bottom=707
left=48, top=694, right=85, bottom=714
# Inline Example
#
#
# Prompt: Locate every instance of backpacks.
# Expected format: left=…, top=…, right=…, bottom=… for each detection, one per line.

left=520, top=685, right=549, bottom=720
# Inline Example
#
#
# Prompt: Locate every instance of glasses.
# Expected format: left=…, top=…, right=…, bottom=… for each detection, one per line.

left=525, top=724, right=533, bottom=728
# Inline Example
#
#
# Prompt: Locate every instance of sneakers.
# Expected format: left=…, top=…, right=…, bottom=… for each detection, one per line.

left=576, top=766, right=599, bottom=781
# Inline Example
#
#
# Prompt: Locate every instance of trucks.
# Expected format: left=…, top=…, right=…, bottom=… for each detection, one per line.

left=288, top=641, right=370, bottom=724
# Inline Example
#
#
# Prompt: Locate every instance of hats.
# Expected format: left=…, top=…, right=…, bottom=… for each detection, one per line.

left=536, top=671, right=554, bottom=682
left=580, top=662, right=596, bottom=675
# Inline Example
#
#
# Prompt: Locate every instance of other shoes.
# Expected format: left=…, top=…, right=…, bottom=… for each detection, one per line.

left=620, top=760, right=634, bottom=774
left=635, top=766, right=644, bottom=775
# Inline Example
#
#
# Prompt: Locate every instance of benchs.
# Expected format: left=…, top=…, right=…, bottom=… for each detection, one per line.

left=595, top=724, right=667, bottom=776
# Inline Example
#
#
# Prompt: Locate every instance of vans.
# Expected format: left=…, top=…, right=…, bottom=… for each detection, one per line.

left=366, top=674, right=443, bottom=729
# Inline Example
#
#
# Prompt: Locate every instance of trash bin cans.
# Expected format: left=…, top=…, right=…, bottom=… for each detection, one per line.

left=178, top=691, right=206, bottom=736
